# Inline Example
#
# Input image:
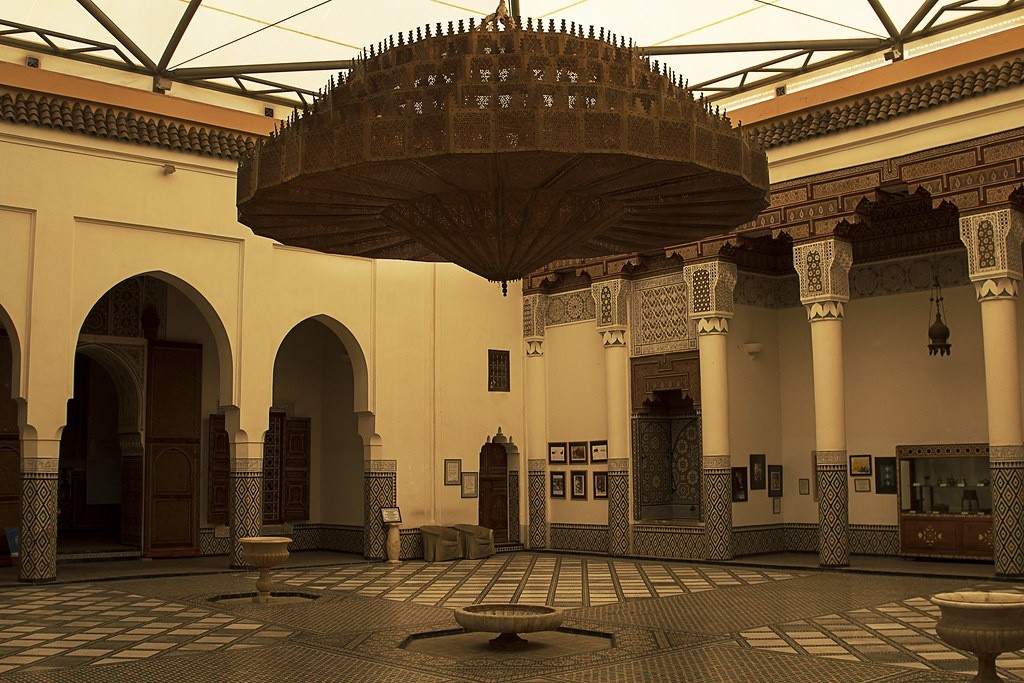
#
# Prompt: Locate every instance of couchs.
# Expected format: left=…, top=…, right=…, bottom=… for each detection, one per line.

left=420, top=526, right=463, bottom=561
left=454, top=524, right=496, bottom=560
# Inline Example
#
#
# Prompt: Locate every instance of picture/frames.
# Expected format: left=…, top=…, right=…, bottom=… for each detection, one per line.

left=380, top=507, right=403, bottom=523
left=732, top=467, right=748, bottom=503
left=461, top=472, right=478, bottom=498
left=750, top=454, right=766, bottom=490
left=569, top=441, right=588, bottom=463
left=768, top=465, right=809, bottom=514
left=550, top=471, right=565, bottom=497
left=445, top=459, right=462, bottom=485
left=849, top=455, right=898, bottom=494
left=590, top=440, right=608, bottom=463
left=549, top=442, right=567, bottom=463
left=571, top=470, right=587, bottom=498
left=593, top=471, right=609, bottom=498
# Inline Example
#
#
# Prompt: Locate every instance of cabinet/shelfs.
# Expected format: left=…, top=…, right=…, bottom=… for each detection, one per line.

left=895, top=443, right=993, bottom=561
